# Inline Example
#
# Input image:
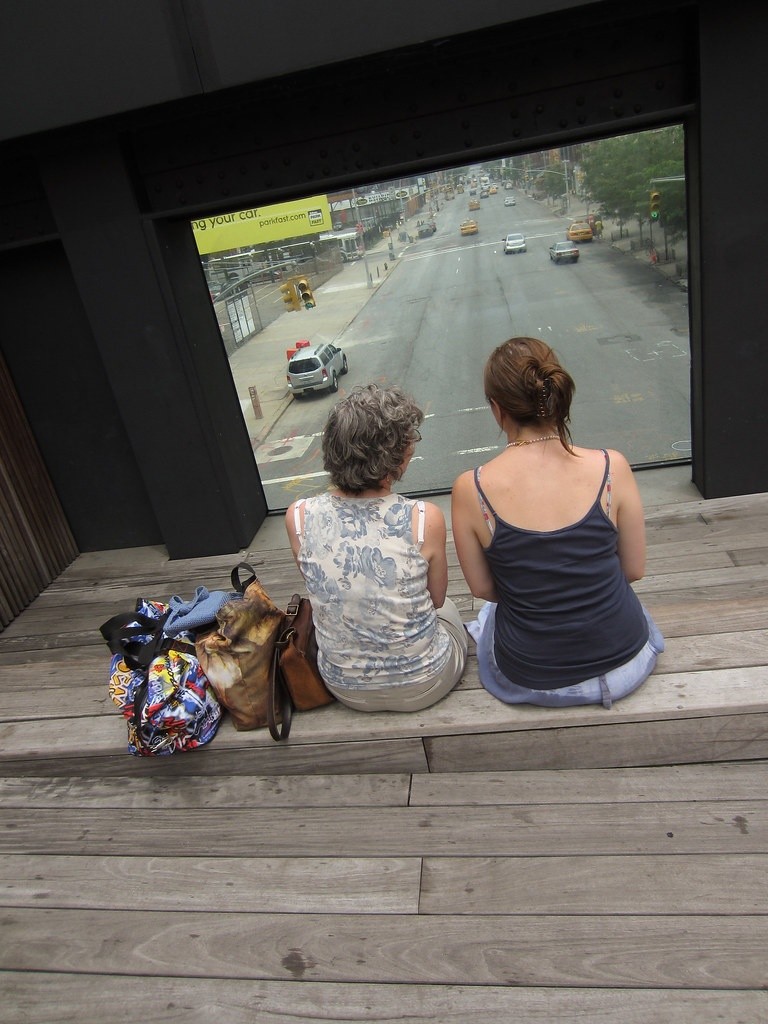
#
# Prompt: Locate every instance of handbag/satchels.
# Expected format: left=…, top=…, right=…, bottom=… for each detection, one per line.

left=100, top=562, right=333, bottom=756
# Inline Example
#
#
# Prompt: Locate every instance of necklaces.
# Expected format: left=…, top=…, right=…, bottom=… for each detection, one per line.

left=505, top=436, right=562, bottom=446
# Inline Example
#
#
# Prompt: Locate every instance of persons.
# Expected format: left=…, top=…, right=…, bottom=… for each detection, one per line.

left=594, top=218, right=604, bottom=243
left=284, top=383, right=470, bottom=714
left=451, top=337, right=666, bottom=710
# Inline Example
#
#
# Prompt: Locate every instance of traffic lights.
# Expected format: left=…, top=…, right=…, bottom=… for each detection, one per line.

left=649, top=191, right=661, bottom=222
left=281, top=280, right=302, bottom=313
left=298, top=279, right=315, bottom=308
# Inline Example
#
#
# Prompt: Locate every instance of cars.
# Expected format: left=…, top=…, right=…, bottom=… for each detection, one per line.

left=461, top=218, right=477, bottom=235
left=549, top=241, right=580, bottom=263
left=425, top=221, right=437, bottom=231
left=419, top=224, right=433, bottom=238
left=502, top=233, right=527, bottom=253
left=565, top=222, right=593, bottom=242
left=435, top=171, right=518, bottom=212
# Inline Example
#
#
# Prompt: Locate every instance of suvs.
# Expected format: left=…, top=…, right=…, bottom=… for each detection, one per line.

left=286, top=345, right=347, bottom=400
